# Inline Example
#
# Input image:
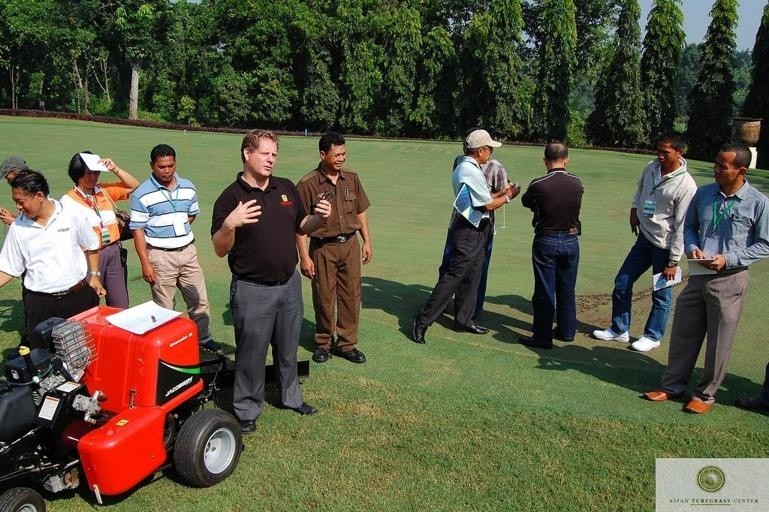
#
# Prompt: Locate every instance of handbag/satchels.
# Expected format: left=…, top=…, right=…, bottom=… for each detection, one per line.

left=116, top=211, right=133, bottom=241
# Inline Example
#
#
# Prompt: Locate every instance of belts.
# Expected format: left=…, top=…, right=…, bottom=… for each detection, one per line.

left=29, top=279, right=86, bottom=297
left=236, top=276, right=289, bottom=286
left=146, top=238, right=195, bottom=252
left=311, top=231, right=356, bottom=243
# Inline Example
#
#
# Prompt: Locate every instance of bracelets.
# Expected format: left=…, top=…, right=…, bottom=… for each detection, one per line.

left=114, top=167, right=120, bottom=174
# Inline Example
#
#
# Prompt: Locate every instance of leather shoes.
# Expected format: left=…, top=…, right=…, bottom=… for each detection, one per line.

left=735, top=398, right=769, bottom=410
left=278, top=400, right=316, bottom=414
left=334, top=348, right=366, bottom=363
left=203, top=341, right=219, bottom=351
left=413, top=315, right=427, bottom=344
left=312, top=348, right=328, bottom=362
left=552, top=330, right=574, bottom=341
left=519, top=335, right=552, bottom=349
left=240, top=420, right=256, bottom=432
left=685, top=399, right=710, bottom=413
left=456, top=323, right=489, bottom=334
left=644, top=388, right=671, bottom=401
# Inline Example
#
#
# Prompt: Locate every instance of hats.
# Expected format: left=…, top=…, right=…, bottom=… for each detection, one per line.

left=465, top=129, right=502, bottom=148
left=0, top=157, right=26, bottom=178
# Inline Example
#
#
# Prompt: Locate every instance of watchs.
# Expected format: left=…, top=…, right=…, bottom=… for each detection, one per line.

left=504, top=195, right=511, bottom=204
left=666, top=260, right=678, bottom=267
left=89, top=271, right=102, bottom=278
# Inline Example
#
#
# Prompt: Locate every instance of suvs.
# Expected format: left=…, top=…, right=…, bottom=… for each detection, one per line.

left=0, top=306, right=244, bottom=512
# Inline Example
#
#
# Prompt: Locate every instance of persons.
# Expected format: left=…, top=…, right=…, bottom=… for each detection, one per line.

left=644, top=143, right=769, bottom=414
left=591, top=130, right=698, bottom=352
left=412, top=128, right=520, bottom=344
left=210, top=128, right=332, bottom=433
left=127, top=142, right=219, bottom=354
left=521, top=143, right=584, bottom=349
left=65, top=150, right=140, bottom=309
left=0, top=156, right=30, bottom=225
left=296, top=133, right=373, bottom=363
left=0, top=170, right=106, bottom=348
left=440, top=127, right=512, bottom=321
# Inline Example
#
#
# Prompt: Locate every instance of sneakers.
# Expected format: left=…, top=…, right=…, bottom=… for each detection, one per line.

left=632, top=337, right=661, bottom=351
left=593, top=330, right=630, bottom=343
